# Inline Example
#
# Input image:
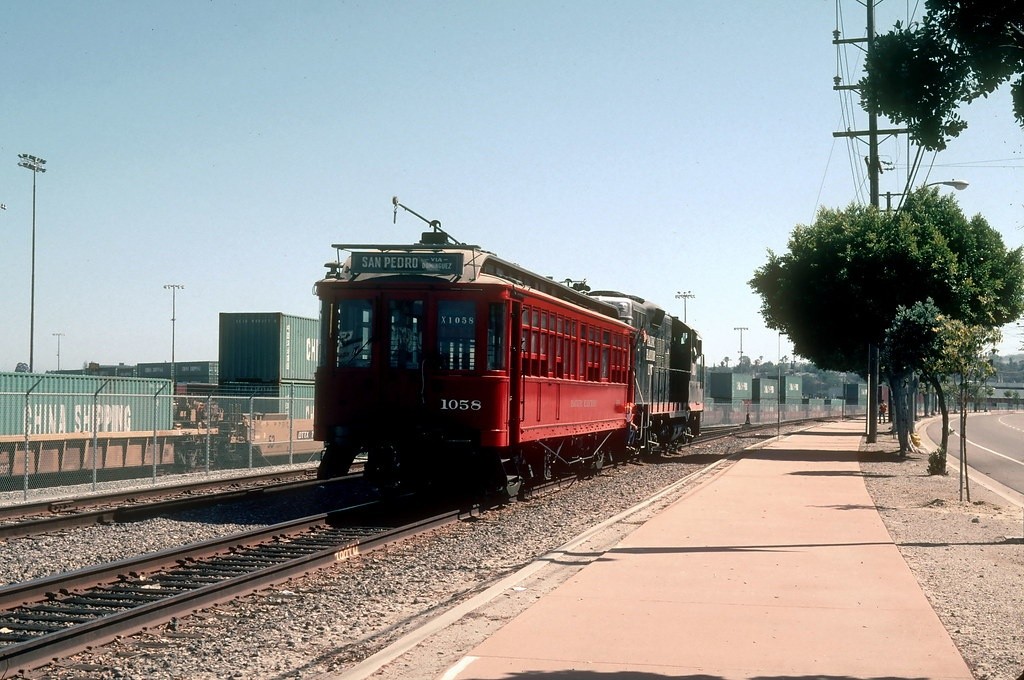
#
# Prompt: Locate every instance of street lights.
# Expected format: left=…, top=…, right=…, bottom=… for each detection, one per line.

left=164, top=285, right=184, bottom=379
left=869, top=180, right=969, bottom=443
left=675, top=291, right=695, bottom=324
left=53, top=333, right=65, bottom=370
left=778, top=332, right=788, bottom=431
left=17, top=153, right=47, bottom=374
left=734, top=327, right=748, bottom=373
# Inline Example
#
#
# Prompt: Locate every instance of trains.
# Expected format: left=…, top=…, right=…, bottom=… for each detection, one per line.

left=313, top=197, right=705, bottom=504
